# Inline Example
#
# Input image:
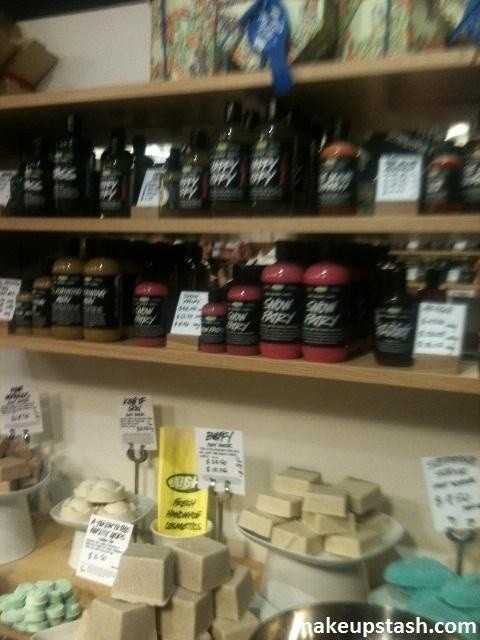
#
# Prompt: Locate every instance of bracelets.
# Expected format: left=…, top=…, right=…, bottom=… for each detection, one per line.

left=247, top=600, right=465, bottom=639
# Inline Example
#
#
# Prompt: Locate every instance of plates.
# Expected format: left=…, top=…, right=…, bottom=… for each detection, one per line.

left=0, top=6, right=467, bottom=379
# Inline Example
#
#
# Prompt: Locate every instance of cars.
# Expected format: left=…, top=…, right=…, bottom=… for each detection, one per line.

left=149, top=518, right=214, bottom=548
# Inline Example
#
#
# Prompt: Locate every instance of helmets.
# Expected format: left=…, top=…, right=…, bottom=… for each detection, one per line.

left=234, top=511, right=404, bottom=565
left=0, top=462, right=52, bottom=498
left=27, top=589, right=280, bottom=640
left=49, top=493, right=155, bottom=532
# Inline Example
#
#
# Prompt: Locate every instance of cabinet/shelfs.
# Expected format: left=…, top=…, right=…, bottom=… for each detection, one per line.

left=248, top=96, right=291, bottom=213
left=81, top=129, right=95, bottom=213
left=284, top=132, right=308, bottom=216
left=32, top=266, right=51, bottom=336
left=21, top=137, right=51, bottom=215
left=225, top=266, right=262, bottom=356
left=84, top=239, right=122, bottom=341
left=129, top=134, right=154, bottom=207
left=316, top=116, right=358, bottom=215
left=113, top=238, right=209, bottom=346
left=372, top=266, right=418, bottom=369
left=423, top=139, right=459, bottom=212
left=259, top=240, right=303, bottom=360
left=98, top=130, right=132, bottom=217
left=447, top=267, right=479, bottom=364
left=51, top=114, right=90, bottom=218
left=208, top=97, right=248, bottom=215
left=200, top=289, right=227, bottom=353
left=414, top=268, right=448, bottom=304
left=300, top=239, right=384, bottom=363
left=14, top=281, right=31, bottom=336
left=175, top=127, right=212, bottom=215
left=460, top=128, right=479, bottom=211
left=50, top=240, right=85, bottom=341
